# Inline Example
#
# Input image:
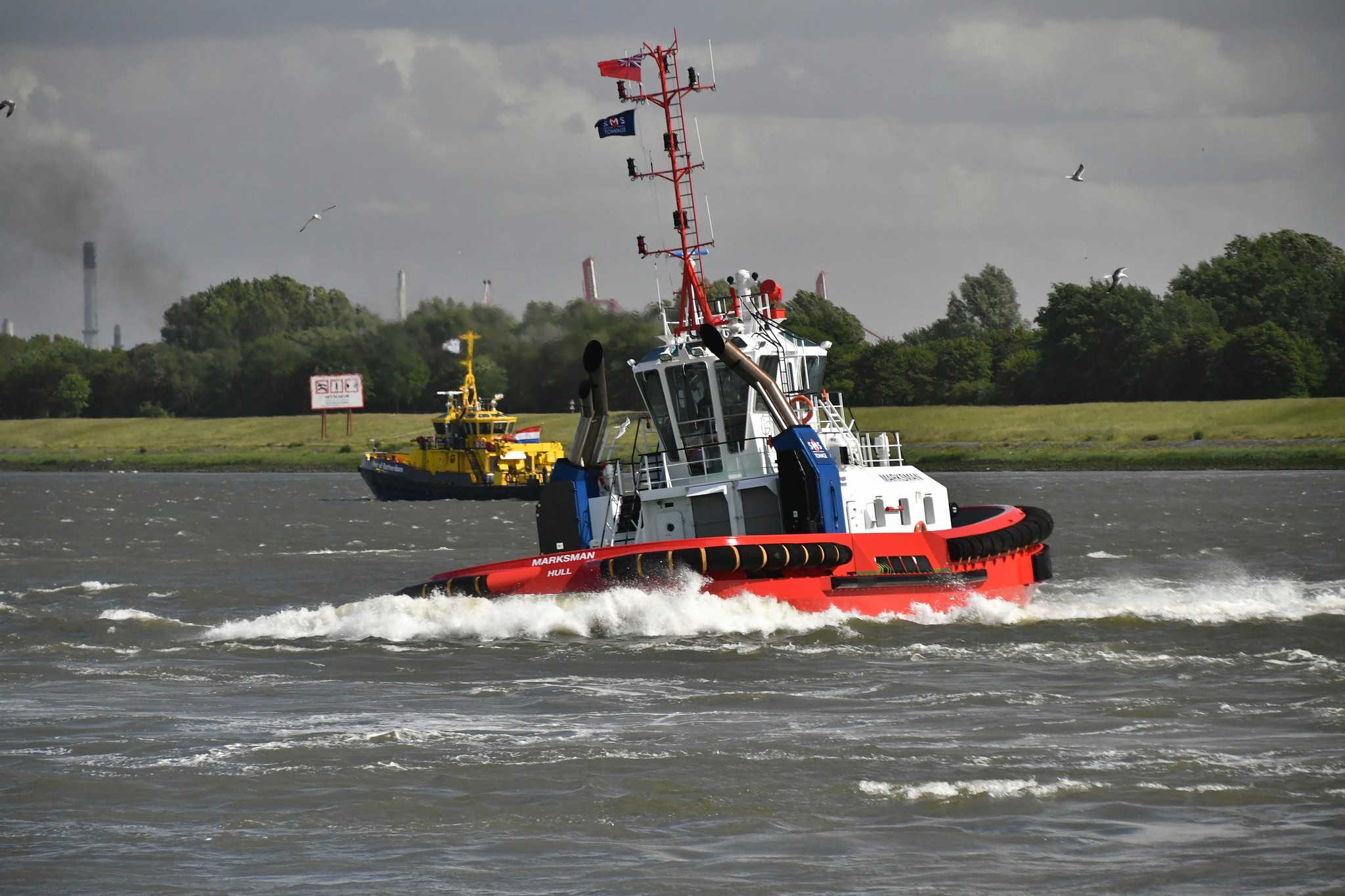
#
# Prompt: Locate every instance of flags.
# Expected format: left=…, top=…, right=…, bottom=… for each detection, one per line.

left=598, top=53, right=642, bottom=82
left=595, top=109, right=635, bottom=138
left=442, top=339, right=460, bottom=354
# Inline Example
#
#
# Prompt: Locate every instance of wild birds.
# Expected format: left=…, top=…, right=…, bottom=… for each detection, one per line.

left=298, top=204, right=337, bottom=233
left=0, top=98, right=16, bottom=119
left=1065, top=163, right=1086, bottom=183
left=1103, top=265, right=1131, bottom=294
left=611, top=416, right=632, bottom=440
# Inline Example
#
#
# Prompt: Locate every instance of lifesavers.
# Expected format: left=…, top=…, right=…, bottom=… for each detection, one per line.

left=505, top=474, right=512, bottom=483
left=914, top=521, right=927, bottom=532
left=599, top=462, right=616, bottom=491
left=789, top=395, right=814, bottom=425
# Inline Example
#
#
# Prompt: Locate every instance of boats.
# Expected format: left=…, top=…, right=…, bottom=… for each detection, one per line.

left=356, top=329, right=563, bottom=501
left=396, top=26, right=1053, bottom=618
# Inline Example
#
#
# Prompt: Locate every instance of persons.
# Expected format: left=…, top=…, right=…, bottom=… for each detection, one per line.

left=698, top=390, right=713, bottom=419
